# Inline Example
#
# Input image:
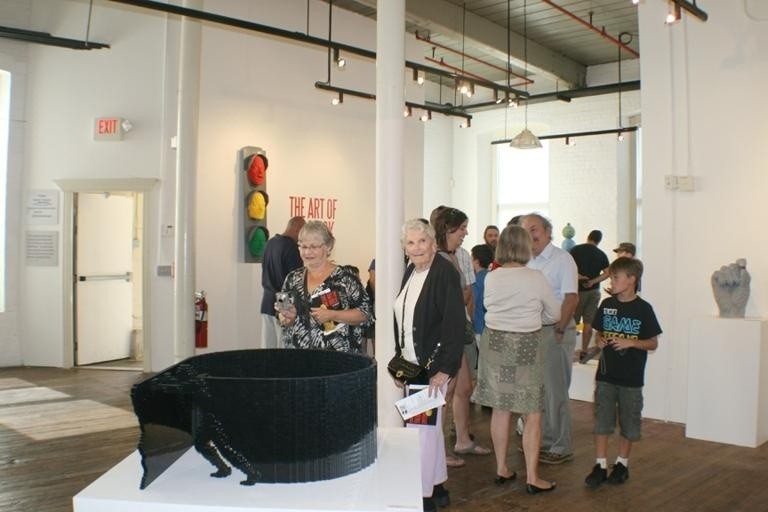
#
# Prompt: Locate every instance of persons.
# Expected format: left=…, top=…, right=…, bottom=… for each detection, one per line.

left=472, top=245, right=495, bottom=414
left=366, top=259, right=375, bottom=355
left=434, top=208, right=492, bottom=467
left=392, top=218, right=466, bottom=511
left=569, top=230, right=611, bottom=364
left=430, top=204, right=477, bottom=444
left=520, top=214, right=579, bottom=465
left=484, top=225, right=499, bottom=250
left=584, top=256, right=663, bottom=487
left=712, top=259, right=751, bottom=318
left=507, top=215, right=523, bottom=226
left=603, top=243, right=642, bottom=296
left=275, top=220, right=375, bottom=355
left=260, top=216, right=306, bottom=349
left=470, top=225, right=561, bottom=494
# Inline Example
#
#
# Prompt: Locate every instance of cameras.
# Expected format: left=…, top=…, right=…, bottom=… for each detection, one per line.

left=274, top=294, right=289, bottom=311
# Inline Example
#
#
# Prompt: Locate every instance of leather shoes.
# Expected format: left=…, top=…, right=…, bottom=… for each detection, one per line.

left=494, top=471, right=517, bottom=486
left=527, top=481, right=556, bottom=493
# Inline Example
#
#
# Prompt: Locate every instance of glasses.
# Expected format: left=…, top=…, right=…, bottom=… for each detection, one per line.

left=298, top=243, right=326, bottom=251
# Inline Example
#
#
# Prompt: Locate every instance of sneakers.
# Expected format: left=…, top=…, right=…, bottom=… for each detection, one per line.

left=584, top=463, right=609, bottom=489
left=607, top=461, right=630, bottom=485
left=578, top=350, right=590, bottom=366
left=538, top=451, right=575, bottom=465
left=518, top=441, right=551, bottom=455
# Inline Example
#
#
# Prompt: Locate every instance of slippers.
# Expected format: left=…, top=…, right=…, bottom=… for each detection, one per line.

left=444, top=454, right=466, bottom=469
left=453, top=442, right=492, bottom=455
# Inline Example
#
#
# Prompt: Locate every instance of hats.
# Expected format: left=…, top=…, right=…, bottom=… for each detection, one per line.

left=613, top=242, right=637, bottom=256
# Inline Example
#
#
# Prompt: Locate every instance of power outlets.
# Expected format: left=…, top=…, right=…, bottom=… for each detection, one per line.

left=664, top=175, right=678, bottom=190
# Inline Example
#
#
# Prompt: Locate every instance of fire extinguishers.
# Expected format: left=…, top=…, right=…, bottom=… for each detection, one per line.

left=194, top=290, right=209, bottom=348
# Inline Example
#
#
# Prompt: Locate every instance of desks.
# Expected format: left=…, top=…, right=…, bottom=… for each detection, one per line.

left=71, top=428, right=424, bottom=511
left=685, top=314, right=766, bottom=447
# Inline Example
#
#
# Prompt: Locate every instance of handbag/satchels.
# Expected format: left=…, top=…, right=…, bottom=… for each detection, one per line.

left=387, top=269, right=441, bottom=385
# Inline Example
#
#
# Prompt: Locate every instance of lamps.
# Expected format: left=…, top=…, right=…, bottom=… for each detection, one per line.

left=509, top=0, right=542, bottom=149
